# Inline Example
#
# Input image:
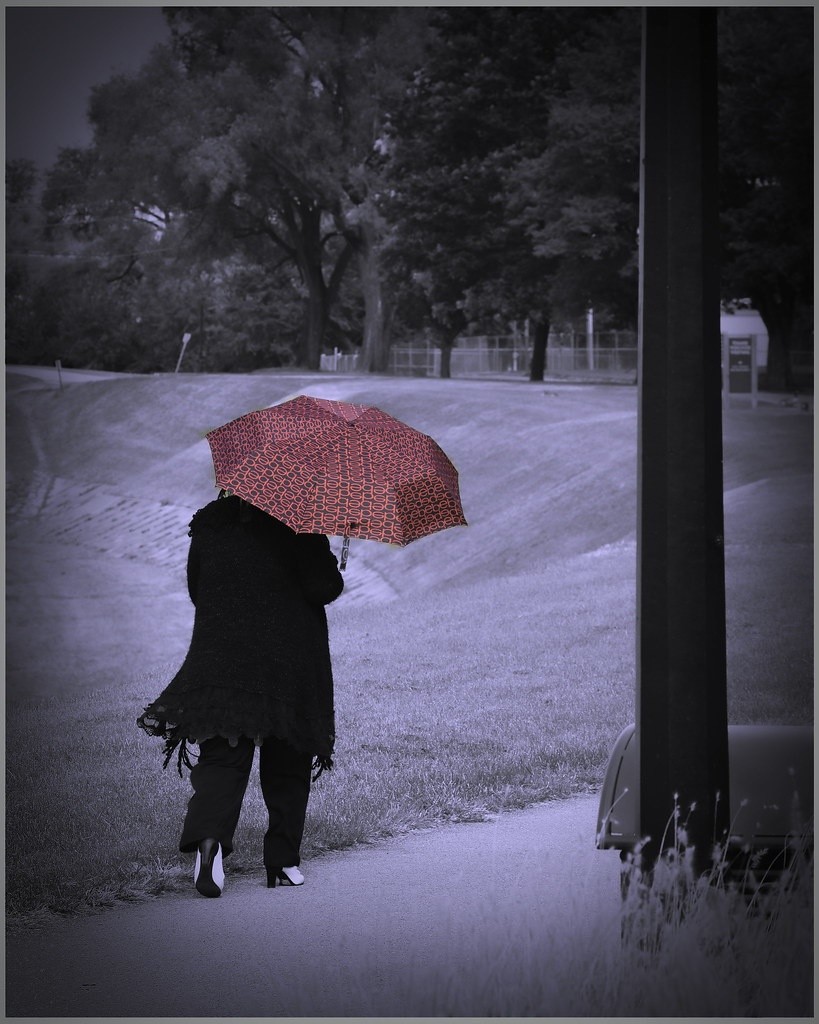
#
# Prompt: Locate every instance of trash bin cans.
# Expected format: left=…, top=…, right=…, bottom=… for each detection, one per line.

left=593, top=718, right=814, bottom=971
left=721, top=298, right=771, bottom=372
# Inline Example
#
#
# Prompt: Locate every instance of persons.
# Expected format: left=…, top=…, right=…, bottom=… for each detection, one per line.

left=136, top=489, right=344, bottom=899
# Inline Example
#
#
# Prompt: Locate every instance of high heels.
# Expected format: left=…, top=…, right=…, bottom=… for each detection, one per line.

left=194, top=838, right=225, bottom=898
left=267, top=866, right=304, bottom=888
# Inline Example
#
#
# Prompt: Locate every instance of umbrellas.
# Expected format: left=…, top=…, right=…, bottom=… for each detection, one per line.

left=205, top=396, right=467, bottom=570
